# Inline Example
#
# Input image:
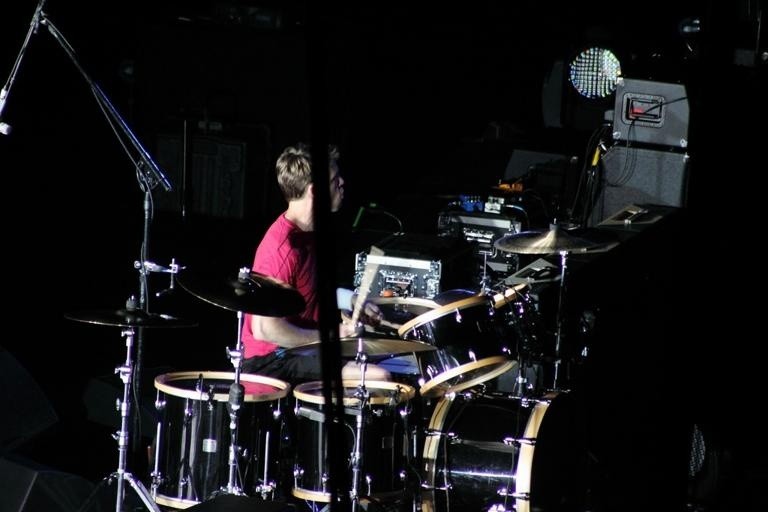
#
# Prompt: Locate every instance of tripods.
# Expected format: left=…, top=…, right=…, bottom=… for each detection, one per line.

left=102, top=328, right=172, bottom=512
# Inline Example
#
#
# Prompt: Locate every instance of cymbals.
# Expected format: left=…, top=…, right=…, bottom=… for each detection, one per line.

left=68, top=297, right=179, bottom=328
left=274, top=338, right=435, bottom=358
left=176, top=264, right=306, bottom=317
left=495, top=222, right=596, bottom=253
left=373, top=295, right=439, bottom=313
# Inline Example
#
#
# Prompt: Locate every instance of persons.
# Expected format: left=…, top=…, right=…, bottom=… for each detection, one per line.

left=241, top=139, right=390, bottom=379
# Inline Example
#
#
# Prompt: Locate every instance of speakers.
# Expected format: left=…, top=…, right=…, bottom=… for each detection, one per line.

left=611, top=78, right=691, bottom=147
left=178, top=494, right=300, bottom=512
left=602, top=145, right=691, bottom=226
left=0, top=453, right=101, bottom=512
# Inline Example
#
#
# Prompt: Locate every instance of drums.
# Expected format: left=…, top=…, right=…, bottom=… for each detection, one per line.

left=398, top=295, right=521, bottom=398
left=414, top=392, right=553, bottom=512
left=293, top=378, right=416, bottom=506
left=150, top=372, right=293, bottom=509
